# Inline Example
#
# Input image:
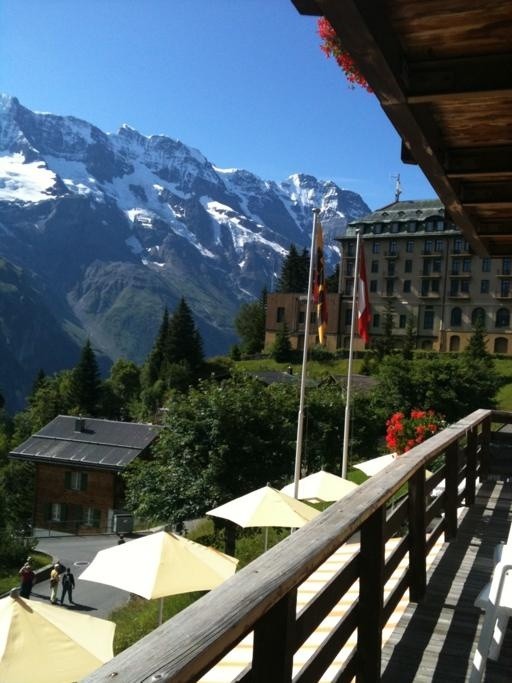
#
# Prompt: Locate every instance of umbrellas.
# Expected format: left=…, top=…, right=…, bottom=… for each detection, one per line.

left=204, top=480, right=323, bottom=550
left=351, top=451, right=435, bottom=482
left=0, top=587, right=117, bottom=681
left=76, top=524, right=241, bottom=626
left=276, top=467, right=360, bottom=503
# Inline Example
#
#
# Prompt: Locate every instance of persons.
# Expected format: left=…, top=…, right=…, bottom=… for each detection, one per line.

left=49, top=562, right=61, bottom=604
left=18, top=560, right=35, bottom=599
left=118, top=533, right=125, bottom=545
left=60, top=566, right=75, bottom=603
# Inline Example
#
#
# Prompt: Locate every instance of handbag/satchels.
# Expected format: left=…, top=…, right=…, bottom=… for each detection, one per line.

left=50, top=578, right=58, bottom=587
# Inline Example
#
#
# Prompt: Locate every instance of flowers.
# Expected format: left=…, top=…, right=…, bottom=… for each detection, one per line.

left=385, top=408, right=466, bottom=468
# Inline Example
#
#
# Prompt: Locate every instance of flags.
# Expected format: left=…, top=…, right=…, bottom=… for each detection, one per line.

left=314, top=213, right=329, bottom=349
left=355, top=234, right=374, bottom=347
